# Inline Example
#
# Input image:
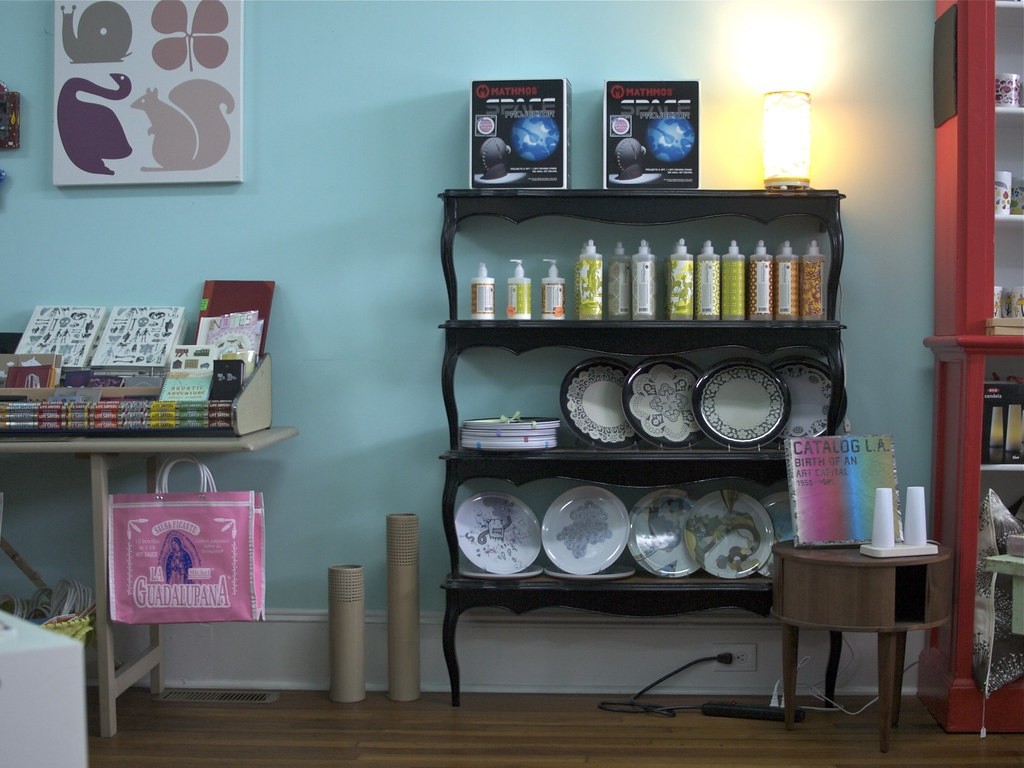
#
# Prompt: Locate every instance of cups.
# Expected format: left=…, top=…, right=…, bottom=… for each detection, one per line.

left=994, top=170, right=1024, bottom=214
left=994, top=283, right=1024, bottom=318
left=995, top=73, right=1024, bottom=107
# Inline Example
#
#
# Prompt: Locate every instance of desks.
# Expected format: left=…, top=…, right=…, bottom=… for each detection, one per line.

left=0, top=426, right=301, bottom=736
left=769, top=539, right=954, bottom=755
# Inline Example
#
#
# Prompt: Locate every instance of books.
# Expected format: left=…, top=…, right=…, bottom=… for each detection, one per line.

left=0, top=281, right=276, bottom=402
left=784, top=434, right=904, bottom=547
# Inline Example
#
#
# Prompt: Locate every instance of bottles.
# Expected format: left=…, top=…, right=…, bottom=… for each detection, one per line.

left=574, top=239, right=826, bottom=322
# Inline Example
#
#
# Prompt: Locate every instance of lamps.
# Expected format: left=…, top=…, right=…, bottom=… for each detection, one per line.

left=763, top=91, right=811, bottom=188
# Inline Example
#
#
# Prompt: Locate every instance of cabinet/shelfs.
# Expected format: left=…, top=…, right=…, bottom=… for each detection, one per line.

left=923, top=0, right=1024, bottom=734
left=436, top=187, right=849, bottom=710
left=0, top=353, right=273, bottom=438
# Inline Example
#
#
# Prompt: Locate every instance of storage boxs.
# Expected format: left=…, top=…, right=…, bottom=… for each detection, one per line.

left=602, top=80, right=700, bottom=189
left=981, top=379, right=1024, bottom=465
left=470, top=78, right=572, bottom=189
left=985, top=318, right=1024, bottom=336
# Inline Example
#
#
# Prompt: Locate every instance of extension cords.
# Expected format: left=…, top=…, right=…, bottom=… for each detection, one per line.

left=701, top=701, right=805, bottom=722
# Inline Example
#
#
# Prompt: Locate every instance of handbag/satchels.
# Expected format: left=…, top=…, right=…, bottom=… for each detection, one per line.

left=108, top=453, right=266, bottom=625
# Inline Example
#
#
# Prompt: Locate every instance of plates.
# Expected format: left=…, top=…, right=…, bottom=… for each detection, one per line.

left=452, top=484, right=792, bottom=579
left=459, top=415, right=561, bottom=451
left=766, top=355, right=847, bottom=448
left=689, top=358, right=791, bottom=449
left=559, top=356, right=642, bottom=449
left=619, top=355, right=705, bottom=449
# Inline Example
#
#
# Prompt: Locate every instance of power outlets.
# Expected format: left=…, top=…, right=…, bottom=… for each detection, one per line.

left=713, top=644, right=758, bottom=672
